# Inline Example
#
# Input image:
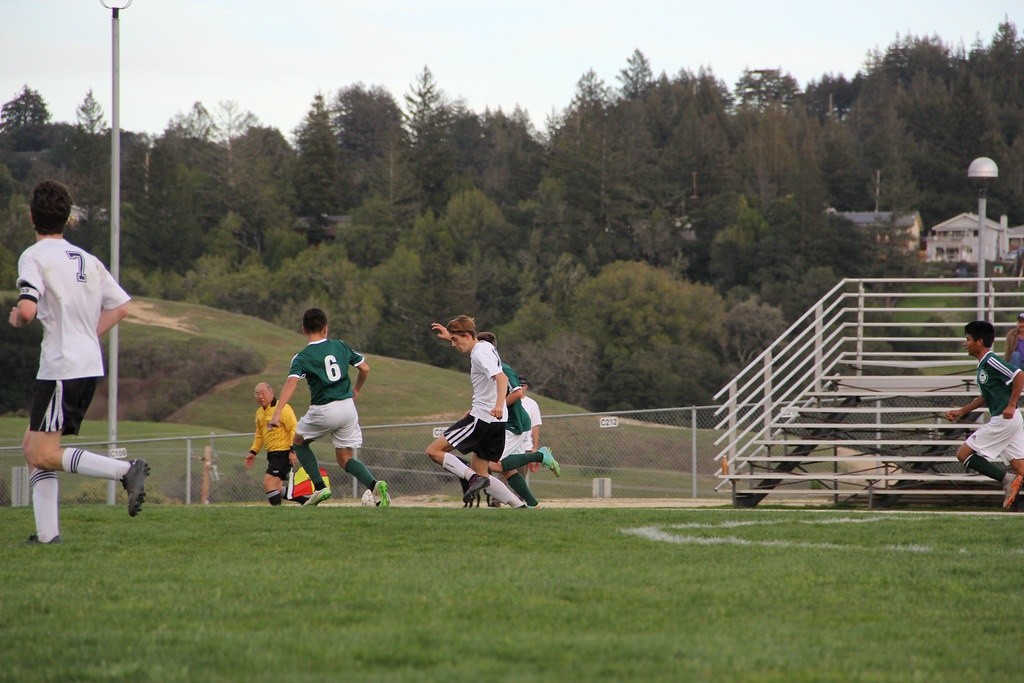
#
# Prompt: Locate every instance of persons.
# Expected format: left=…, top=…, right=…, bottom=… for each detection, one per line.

left=424, top=315, right=561, bottom=510
left=268, top=308, right=391, bottom=507
left=9, top=180, right=149, bottom=545
left=243, top=382, right=309, bottom=506
left=946, top=311, right=1024, bottom=508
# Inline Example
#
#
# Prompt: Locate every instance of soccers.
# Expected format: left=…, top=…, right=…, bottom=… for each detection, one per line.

left=361, top=489, right=392, bottom=510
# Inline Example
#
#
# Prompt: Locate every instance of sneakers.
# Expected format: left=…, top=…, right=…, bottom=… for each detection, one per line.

left=528, top=503, right=542, bottom=509
left=120, top=459, right=150, bottom=517
left=463, top=476, right=490, bottom=503
left=538, top=446, right=562, bottom=477
left=303, top=487, right=332, bottom=507
left=1003, top=474, right=1023, bottom=508
left=373, top=481, right=389, bottom=507
left=21, top=533, right=61, bottom=544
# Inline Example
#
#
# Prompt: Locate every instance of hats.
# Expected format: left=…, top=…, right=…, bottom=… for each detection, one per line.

left=1017, top=312, right=1024, bottom=320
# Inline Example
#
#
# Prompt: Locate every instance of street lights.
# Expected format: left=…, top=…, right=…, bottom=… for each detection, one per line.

left=967, top=156, right=1002, bottom=323
